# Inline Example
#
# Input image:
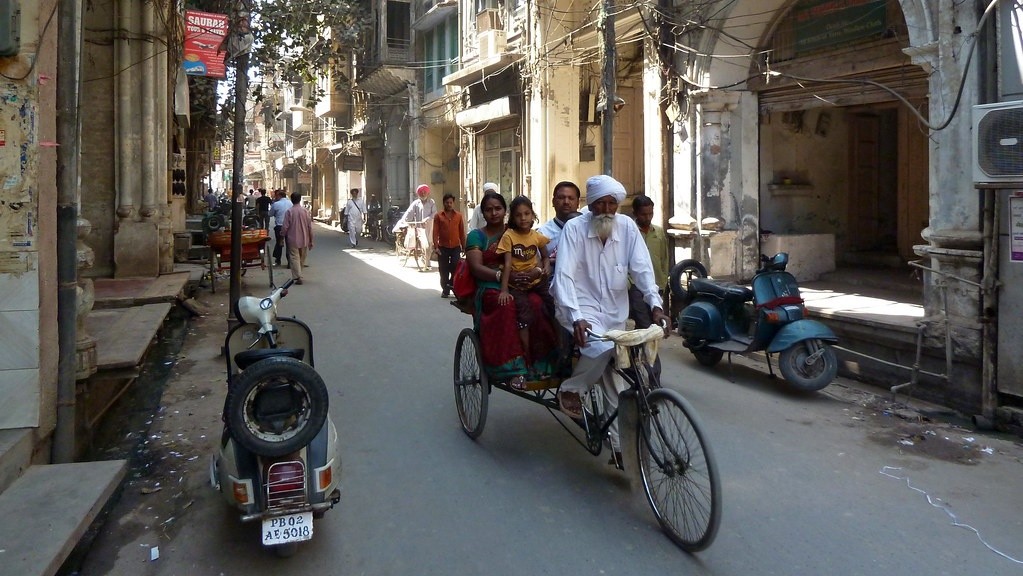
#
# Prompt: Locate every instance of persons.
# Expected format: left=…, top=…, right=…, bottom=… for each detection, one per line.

left=204, top=188, right=226, bottom=212
left=244, top=187, right=273, bottom=235
left=468, top=182, right=498, bottom=233
left=628, top=196, right=669, bottom=390
left=466, top=192, right=550, bottom=393
left=268, top=187, right=294, bottom=268
left=402, top=185, right=438, bottom=272
left=279, top=192, right=313, bottom=284
left=433, top=194, right=465, bottom=298
left=536, top=181, right=581, bottom=258
left=343, top=189, right=367, bottom=248
left=549, top=175, right=671, bottom=464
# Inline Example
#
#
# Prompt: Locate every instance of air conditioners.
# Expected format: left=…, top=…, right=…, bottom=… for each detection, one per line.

left=477, top=30, right=507, bottom=61
left=475, top=8, right=505, bottom=32
left=971, top=100, right=1023, bottom=183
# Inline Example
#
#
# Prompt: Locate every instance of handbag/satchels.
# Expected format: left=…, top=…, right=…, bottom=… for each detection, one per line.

left=452, top=257, right=476, bottom=307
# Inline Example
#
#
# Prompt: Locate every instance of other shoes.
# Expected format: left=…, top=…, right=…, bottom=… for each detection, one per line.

left=272, top=263, right=282, bottom=267
left=295, top=279, right=303, bottom=285
left=441, top=291, right=449, bottom=298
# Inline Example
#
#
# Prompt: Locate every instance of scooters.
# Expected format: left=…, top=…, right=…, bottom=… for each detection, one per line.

left=668, top=251, right=841, bottom=392
left=209, top=275, right=343, bottom=557
left=337, top=206, right=351, bottom=233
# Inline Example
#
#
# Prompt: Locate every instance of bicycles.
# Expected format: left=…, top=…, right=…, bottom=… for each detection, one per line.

left=396, top=219, right=430, bottom=273
left=362, top=192, right=406, bottom=248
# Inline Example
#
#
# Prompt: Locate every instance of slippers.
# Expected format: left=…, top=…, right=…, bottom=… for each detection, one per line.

left=556, top=389, right=584, bottom=420
left=610, top=451, right=625, bottom=472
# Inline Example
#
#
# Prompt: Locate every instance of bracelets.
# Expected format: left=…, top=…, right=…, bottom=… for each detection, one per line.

left=495, top=270, right=502, bottom=282
left=532, top=265, right=542, bottom=278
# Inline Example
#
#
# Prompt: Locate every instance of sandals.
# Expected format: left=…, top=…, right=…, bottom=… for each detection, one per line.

left=509, top=375, right=527, bottom=393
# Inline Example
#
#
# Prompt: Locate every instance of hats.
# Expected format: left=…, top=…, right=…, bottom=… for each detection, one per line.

left=584, top=173, right=629, bottom=209
left=483, top=181, right=501, bottom=194
left=416, top=184, right=430, bottom=195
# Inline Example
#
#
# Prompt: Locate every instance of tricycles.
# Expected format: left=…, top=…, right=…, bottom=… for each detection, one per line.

left=205, top=211, right=274, bottom=294
left=450, top=301, right=725, bottom=551
